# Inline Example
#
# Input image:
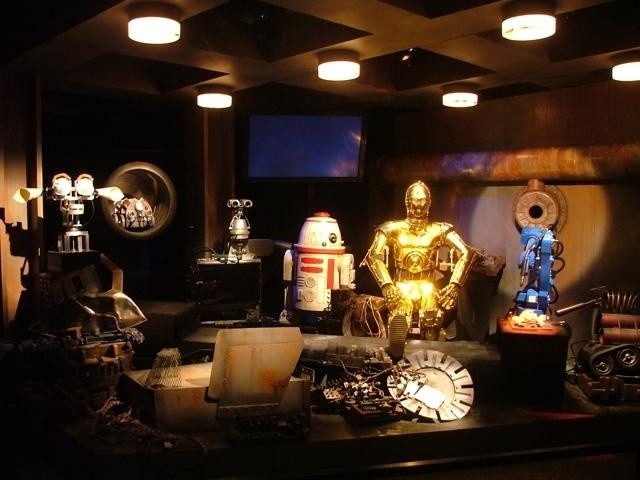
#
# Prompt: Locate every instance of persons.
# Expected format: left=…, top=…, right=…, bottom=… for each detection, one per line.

left=365, top=181, right=476, bottom=359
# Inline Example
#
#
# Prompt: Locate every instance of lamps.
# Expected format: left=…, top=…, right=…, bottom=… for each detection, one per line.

left=608, top=49, right=640, bottom=82
left=500, top=0, right=560, bottom=43
left=315, top=46, right=362, bottom=84
left=193, top=81, right=235, bottom=111
left=123, top=1, right=182, bottom=46
left=439, top=81, right=480, bottom=110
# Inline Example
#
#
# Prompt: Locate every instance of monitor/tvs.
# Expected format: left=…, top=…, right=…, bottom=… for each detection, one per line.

left=236, top=99, right=369, bottom=190
